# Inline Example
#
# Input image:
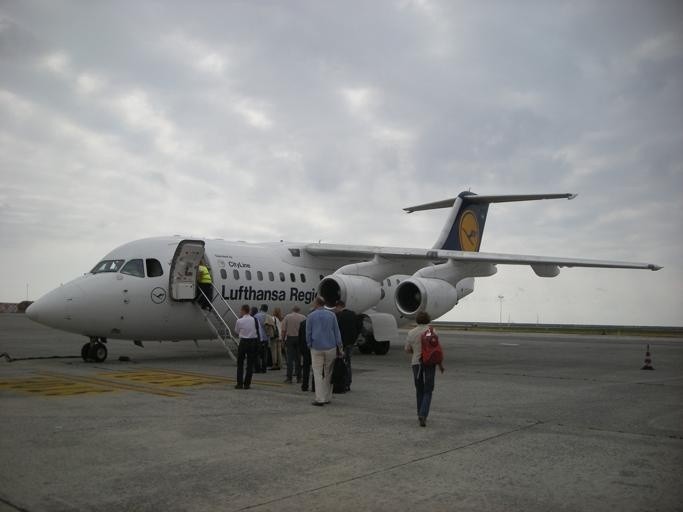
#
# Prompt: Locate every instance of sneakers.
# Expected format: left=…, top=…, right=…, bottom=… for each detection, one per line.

left=311, top=401, right=329, bottom=406
left=418, top=415, right=427, bottom=426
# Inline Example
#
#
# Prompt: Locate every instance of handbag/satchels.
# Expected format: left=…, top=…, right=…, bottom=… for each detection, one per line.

left=421, top=327, right=442, bottom=366
left=331, top=357, right=347, bottom=393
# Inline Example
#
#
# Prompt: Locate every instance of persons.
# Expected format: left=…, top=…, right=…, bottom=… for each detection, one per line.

left=404, top=311, right=445, bottom=427
left=196, top=258, right=213, bottom=312
left=233, top=296, right=364, bottom=407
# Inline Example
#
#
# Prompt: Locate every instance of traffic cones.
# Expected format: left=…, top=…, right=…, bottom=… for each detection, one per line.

left=639, top=343, right=657, bottom=370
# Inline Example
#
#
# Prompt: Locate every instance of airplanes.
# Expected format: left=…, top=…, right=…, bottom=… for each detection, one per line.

left=25, top=191, right=663, bottom=364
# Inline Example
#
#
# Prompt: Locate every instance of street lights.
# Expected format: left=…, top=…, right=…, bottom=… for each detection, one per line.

left=498, top=296, right=504, bottom=323
